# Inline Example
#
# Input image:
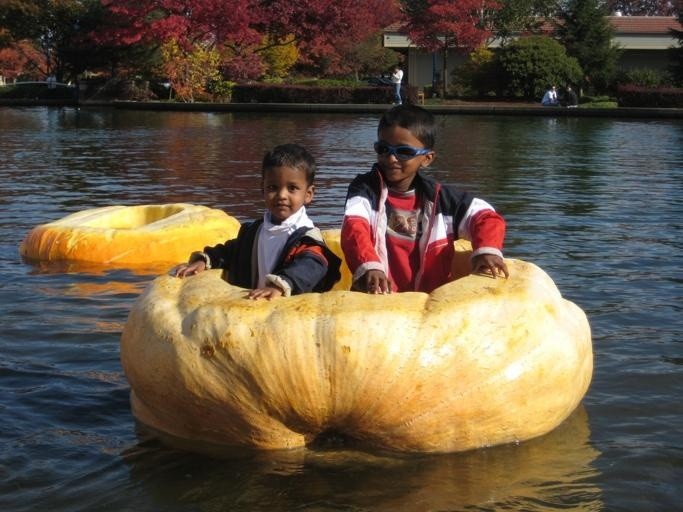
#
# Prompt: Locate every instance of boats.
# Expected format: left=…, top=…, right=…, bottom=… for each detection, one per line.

left=121, top=229, right=594, bottom=464
left=23, top=201, right=243, bottom=275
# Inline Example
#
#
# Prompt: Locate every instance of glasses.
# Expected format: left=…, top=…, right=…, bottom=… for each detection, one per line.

left=374, top=141, right=430, bottom=161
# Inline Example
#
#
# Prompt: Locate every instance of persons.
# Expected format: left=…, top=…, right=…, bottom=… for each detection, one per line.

left=540, top=82, right=561, bottom=106
left=563, top=82, right=578, bottom=109
left=339, top=104, right=510, bottom=295
left=391, top=64, right=404, bottom=106
left=174, top=143, right=342, bottom=303
left=45, top=71, right=58, bottom=99
